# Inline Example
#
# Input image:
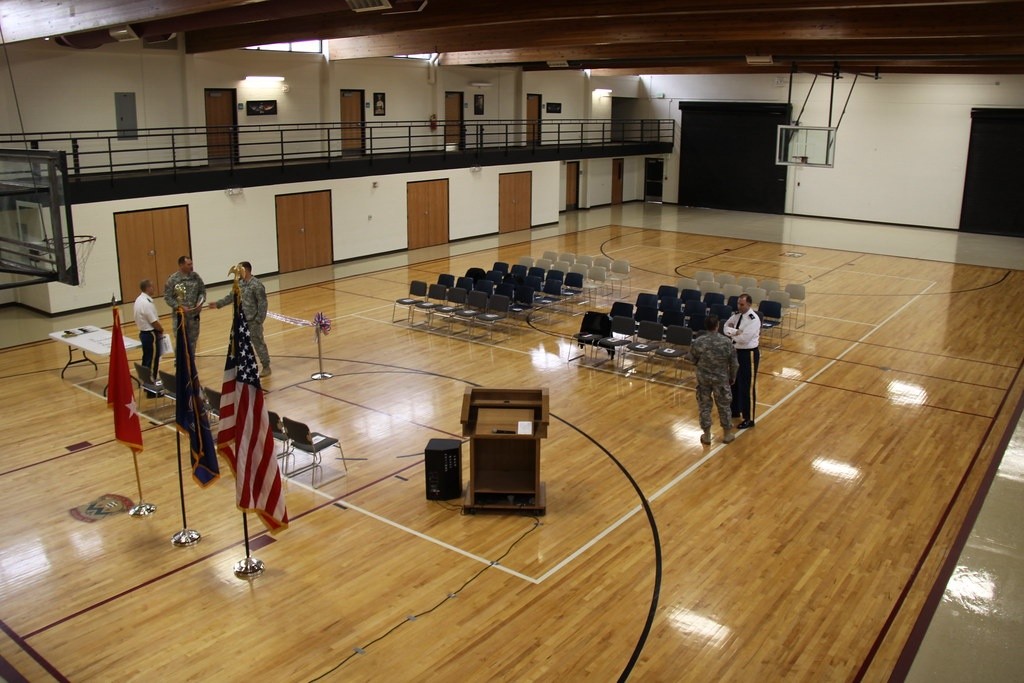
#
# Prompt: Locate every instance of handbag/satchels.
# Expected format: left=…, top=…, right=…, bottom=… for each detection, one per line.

left=160, top=333, right=174, bottom=355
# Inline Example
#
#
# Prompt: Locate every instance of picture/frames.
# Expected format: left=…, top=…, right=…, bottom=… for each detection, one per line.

left=373, top=92, right=386, bottom=116
left=246, top=100, right=278, bottom=116
left=474, top=95, right=484, bottom=115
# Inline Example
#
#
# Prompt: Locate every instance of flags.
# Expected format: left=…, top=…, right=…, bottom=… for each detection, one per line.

left=106, top=307, right=143, bottom=456
left=216, top=281, right=289, bottom=535
left=173, top=309, right=221, bottom=491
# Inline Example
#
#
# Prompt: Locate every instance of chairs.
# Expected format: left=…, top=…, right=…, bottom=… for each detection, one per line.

left=567, top=271, right=806, bottom=392
left=390, top=249, right=632, bottom=343
left=132, top=361, right=348, bottom=489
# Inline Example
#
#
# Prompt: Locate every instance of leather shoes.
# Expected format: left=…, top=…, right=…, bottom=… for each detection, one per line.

left=737, top=419, right=755, bottom=428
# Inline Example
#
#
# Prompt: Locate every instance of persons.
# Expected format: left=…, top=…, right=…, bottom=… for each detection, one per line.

left=207, top=261, right=271, bottom=377
left=164, top=256, right=206, bottom=365
left=133, top=280, right=164, bottom=382
left=723, top=294, right=761, bottom=429
left=689, top=316, right=739, bottom=445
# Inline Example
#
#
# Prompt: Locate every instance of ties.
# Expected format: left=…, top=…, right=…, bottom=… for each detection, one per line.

left=732, top=314, right=743, bottom=344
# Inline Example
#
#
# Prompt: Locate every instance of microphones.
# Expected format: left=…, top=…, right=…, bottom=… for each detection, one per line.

left=492, top=428, right=516, bottom=434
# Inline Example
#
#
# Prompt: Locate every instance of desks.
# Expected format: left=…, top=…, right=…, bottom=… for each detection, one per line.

left=49, top=325, right=143, bottom=398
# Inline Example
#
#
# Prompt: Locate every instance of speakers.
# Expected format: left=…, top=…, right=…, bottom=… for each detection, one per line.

left=425, top=438, right=463, bottom=499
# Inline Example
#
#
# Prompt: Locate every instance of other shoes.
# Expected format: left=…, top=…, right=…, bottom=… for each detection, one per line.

left=259, top=366, right=272, bottom=377
left=701, top=434, right=711, bottom=444
left=144, top=389, right=165, bottom=398
left=723, top=432, right=735, bottom=443
